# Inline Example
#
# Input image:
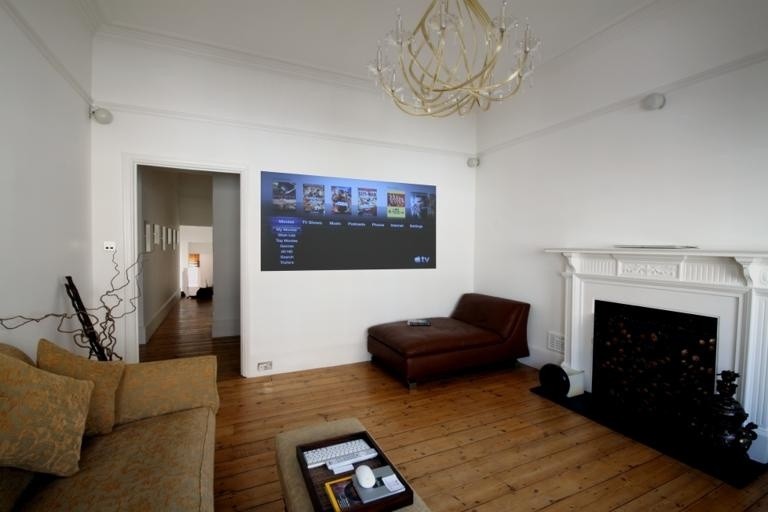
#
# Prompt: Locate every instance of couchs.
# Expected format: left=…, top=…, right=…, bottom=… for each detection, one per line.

left=0, top=340, right=223, bottom=512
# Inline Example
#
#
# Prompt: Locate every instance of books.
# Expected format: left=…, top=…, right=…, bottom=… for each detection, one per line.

left=351, top=465, right=406, bottom=504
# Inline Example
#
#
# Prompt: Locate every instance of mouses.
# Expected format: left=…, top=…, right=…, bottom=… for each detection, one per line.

left=356, top=465, right=376, bottom=489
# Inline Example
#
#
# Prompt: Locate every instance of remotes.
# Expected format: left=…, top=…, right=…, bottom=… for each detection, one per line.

left=326, top=449, right=378, bottom=470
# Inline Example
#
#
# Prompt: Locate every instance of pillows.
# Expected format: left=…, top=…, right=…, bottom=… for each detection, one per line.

left=0, top=352, right=95, bottom=478
left=36, top=336, right=130, bottom=433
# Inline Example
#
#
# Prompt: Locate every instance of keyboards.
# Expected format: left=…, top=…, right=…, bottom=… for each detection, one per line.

left=304, top=439, right=371, bottom=470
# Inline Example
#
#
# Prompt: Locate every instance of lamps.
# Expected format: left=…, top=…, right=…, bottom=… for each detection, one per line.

left=637, top=93, right=667, bottom=114
left=371, top=0, right=544, bottom=125
left=87, top=105, right=114, bottom=126
left=466, top=158, right=483, bottom=168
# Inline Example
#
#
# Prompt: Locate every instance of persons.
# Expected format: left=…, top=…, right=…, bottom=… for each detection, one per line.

left=314, top=189, right=322, bottom=197
left=337, top=189, right=344, bottom=196
left=307, top=187, right=315, bottom=196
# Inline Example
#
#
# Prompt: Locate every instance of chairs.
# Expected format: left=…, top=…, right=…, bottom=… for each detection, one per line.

left=367, top=290, right=532, bottom=390
